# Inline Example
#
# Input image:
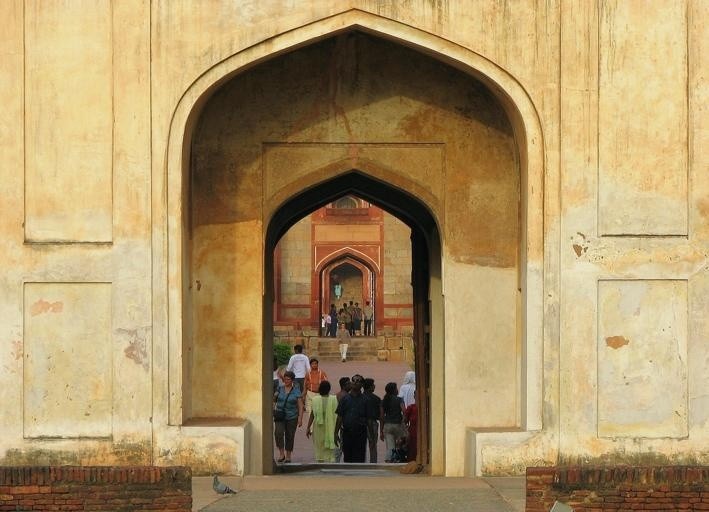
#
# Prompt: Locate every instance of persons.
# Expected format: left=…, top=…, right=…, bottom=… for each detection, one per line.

left=322, top=301, right=373, bottom=336
left=273, top=344, right=417, bottom=463
left=333, top=283, right=341, bottom=301
left=336, top=323, right=351, bottom=362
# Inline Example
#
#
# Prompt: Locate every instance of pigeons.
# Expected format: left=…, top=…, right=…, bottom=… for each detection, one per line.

left=213, top=475, right=237, bottom=494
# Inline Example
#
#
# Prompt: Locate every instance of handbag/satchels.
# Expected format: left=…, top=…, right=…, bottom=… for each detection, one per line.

left=273, top=408, right=284, bottom=421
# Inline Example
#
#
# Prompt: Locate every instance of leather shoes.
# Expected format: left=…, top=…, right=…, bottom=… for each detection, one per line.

left=278, top=457, right=291, bottom=463
